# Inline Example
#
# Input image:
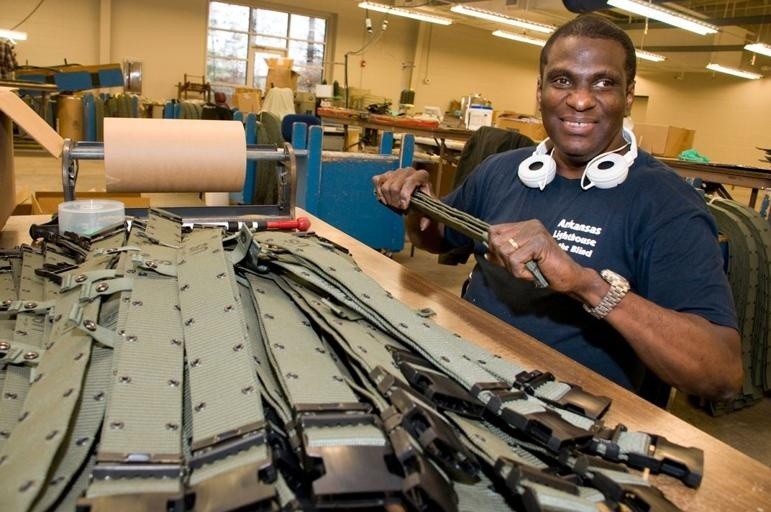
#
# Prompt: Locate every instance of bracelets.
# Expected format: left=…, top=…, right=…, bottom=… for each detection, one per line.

left=582, top=268, right=630, bottom=320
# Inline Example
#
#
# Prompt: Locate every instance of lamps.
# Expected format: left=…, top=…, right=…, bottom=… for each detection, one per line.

left=358, top=1, right=453, bottom=32
left=450, top=4, right=558, bottom=36
left=706, top=59, right=764, bottom=80
left=609, top=0, right=719, bottom=37
left=745, top=40, right=771, bottom=58
left=492, top=27, right=549, bottom=52
left=627, top=19, right=669, bottom=68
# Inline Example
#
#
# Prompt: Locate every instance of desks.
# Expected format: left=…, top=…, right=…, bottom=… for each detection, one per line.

left=0, top=205, right=771, bottom=511
left=322, top=107, right=771, bottom=258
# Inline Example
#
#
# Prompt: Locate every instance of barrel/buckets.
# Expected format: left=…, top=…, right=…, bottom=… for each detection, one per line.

left=469, top=105, right=494, bottom=132
left=58, top=200, right=124, bottom=240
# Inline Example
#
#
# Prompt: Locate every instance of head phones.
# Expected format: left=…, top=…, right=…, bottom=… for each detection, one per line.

left=516, top=126, right=638, bottom=189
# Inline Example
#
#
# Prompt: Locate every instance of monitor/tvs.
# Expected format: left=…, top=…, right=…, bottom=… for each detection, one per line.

left=424, top=106, right=443, bottom=123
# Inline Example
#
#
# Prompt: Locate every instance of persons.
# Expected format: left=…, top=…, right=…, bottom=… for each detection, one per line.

left=371, top=12, right=744, bottom=412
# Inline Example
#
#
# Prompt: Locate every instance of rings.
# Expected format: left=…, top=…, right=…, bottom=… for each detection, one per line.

left=507, top=238, right=521, bottom=249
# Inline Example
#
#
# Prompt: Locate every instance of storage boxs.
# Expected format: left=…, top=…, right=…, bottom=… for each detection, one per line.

left=232, top=58, right=316, bottom=121
left=0, top=87, right=65, bottom=231
